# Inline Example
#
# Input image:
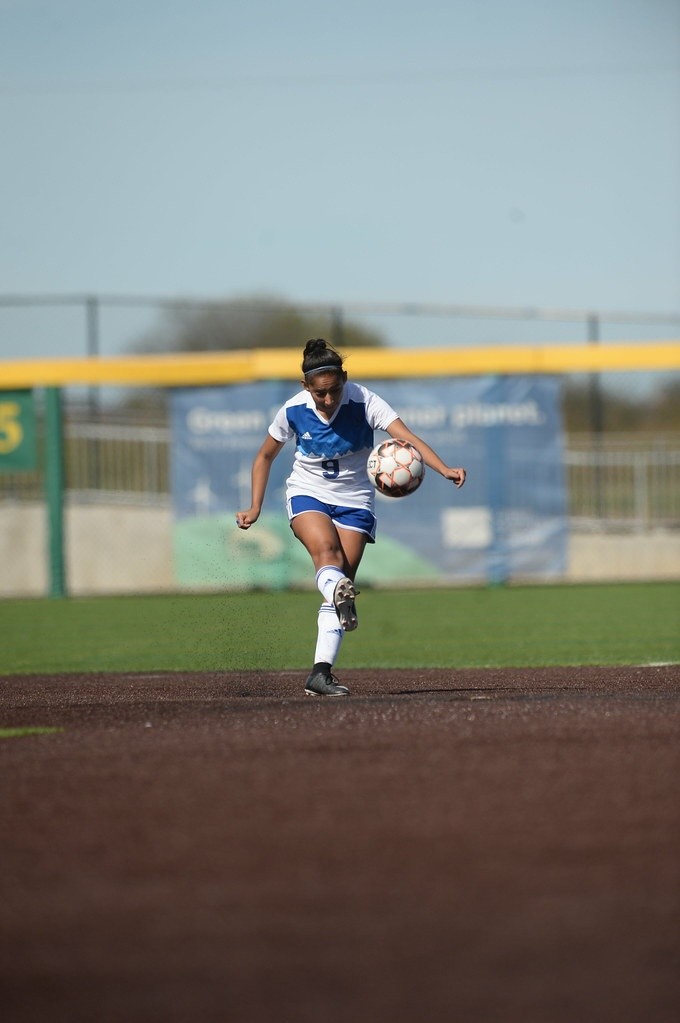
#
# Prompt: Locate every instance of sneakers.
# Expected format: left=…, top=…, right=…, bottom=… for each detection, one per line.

left=333, top=577, right=360, bottom=632
left=304, top=672, right=350, bottom=697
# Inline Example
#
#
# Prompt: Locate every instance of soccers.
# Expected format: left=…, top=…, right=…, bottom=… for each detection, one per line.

left=367, top=436, right=425, bottom=498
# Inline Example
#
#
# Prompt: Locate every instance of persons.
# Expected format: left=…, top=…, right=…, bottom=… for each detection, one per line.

left=235, top=339, right=466, bottom=697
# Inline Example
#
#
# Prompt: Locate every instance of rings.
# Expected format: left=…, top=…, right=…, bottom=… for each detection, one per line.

left=236, top=521, right=239, bottom=524
